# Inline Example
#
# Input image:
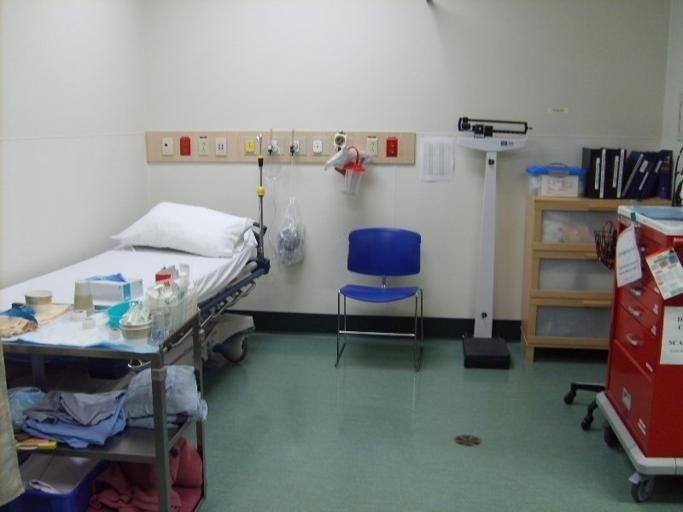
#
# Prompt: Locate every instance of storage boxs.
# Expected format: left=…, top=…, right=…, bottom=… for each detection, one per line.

left=524, top=164, right=586, bottom=199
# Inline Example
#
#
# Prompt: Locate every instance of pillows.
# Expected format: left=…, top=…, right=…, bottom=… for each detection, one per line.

left=110, top=200, right=254, bottom=259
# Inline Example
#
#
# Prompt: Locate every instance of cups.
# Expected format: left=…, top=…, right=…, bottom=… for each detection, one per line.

left=74, top=280, right=93, bottom=316
left=342, top=161, right=364, bottom=198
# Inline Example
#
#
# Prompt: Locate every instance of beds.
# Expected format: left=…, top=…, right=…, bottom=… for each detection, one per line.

left=1, top=201, right=271, bottom=433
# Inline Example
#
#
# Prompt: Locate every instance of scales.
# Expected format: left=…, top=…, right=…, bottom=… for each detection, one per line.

left=452, top=116, right=529, bottom=370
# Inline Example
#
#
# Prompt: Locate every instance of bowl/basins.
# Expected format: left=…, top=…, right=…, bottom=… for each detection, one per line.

left=24, top=290, right=52, bottom=312
left=118, top=319, right=150, bottom=337
left=106, top=301, right=143, bottom=328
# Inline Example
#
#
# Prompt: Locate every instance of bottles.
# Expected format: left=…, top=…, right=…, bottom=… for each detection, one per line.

left=156, top=274, right=174, bottom=305
left=148, top=309, right=165, bottom=344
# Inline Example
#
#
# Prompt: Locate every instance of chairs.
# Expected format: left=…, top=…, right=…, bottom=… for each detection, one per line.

left=334, top=226, right=425, bottom=372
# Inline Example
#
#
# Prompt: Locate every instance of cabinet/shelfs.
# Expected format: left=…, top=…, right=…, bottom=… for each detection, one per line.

left=602, top=206, right=683, bottom=479
left=521, top=192, right=672, bottom=365
left=1, top=312, right=208, bottom=511
